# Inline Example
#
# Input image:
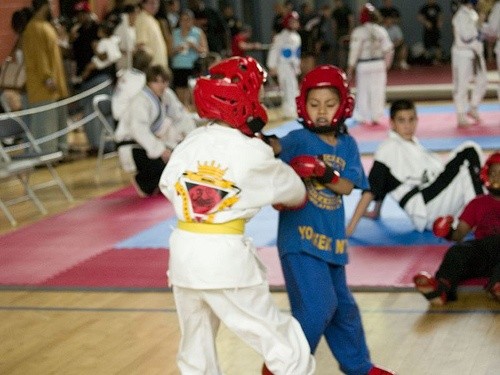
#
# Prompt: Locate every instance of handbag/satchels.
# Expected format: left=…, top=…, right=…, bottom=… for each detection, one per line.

left=0, top=37, right=28, bottom=89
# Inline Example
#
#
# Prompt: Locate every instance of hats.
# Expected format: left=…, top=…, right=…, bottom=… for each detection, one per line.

left=75, top=2, right=93, bottom=13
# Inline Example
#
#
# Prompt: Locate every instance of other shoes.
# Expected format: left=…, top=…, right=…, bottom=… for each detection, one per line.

left=367, top=366, right=394, bottom=375
left=262, top=363, right=273, bottom=375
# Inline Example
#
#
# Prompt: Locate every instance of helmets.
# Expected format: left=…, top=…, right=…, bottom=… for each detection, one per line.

left=282, top=11, right=301, bottom=30
left=360, top=3, right=379, bottom=24
left=193, top=56, right=268, bottom=137
left=480, top=152, right=500, bottom=188
left=295, top=65, right=355, bottom=128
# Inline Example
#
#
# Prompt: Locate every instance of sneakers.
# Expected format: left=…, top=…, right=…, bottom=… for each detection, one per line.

left=400, top=61, right=410, bottom=69
left=413, top=271, right=451, bottom=306
left=87, top=148, right=110, bottom=157
left=130, top=175, right=147, bottom=198
left=484, top=280, right=500, bottom=299
left=458, top=109, right=478, bottom=124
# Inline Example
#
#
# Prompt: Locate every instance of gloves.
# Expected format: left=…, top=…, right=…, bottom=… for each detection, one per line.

left=290, top=155, right=340, bottom=185
left=433, top=216, right=455, bottom=243
left=271, top=191, right=309, bottom=212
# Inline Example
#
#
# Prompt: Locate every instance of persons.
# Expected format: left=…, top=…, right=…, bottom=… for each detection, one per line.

left=0, top=1, right=265, bottom=170
left=413, top=153, right=500, bottom=305
left=346, top=99, right=489, bottom=239
left=262, top=64, right=394, bottom=375
left=115, top=66, right=197, bottom=198
left=159, top=56, right=316, bottom=375
left=451, top=0, right=489, bottom=128
left=347, top=3, right=395, bottom=126
left=273, top=1, right=499, bottom=101
left=266, top=11, right=302, bottom=119
